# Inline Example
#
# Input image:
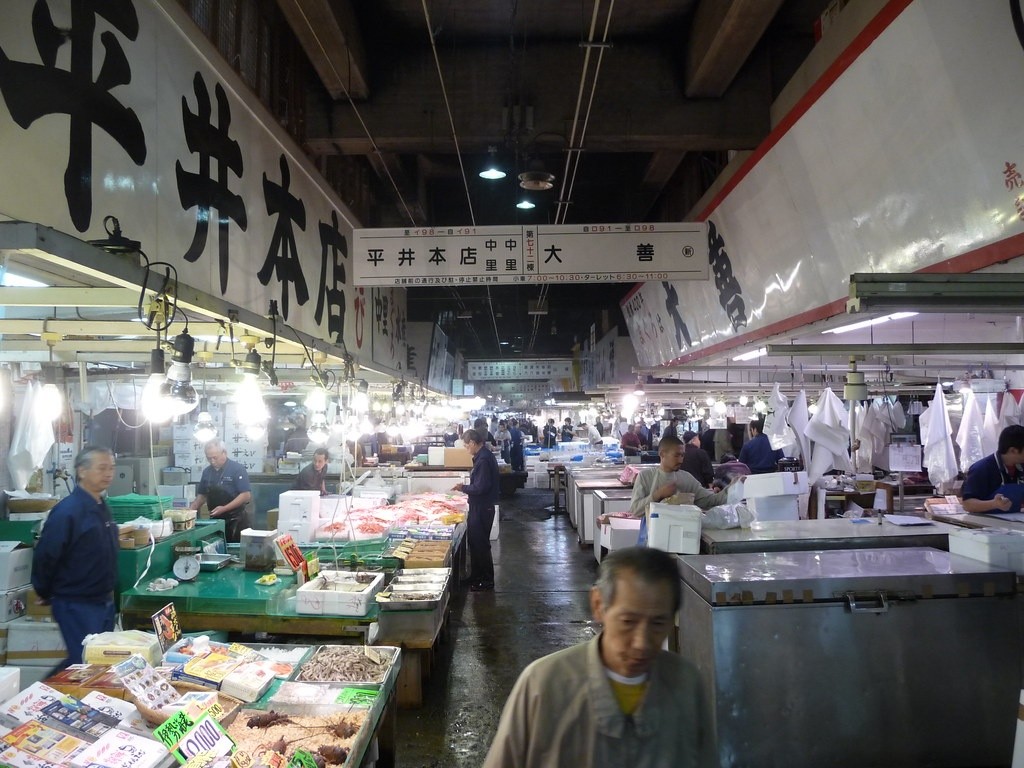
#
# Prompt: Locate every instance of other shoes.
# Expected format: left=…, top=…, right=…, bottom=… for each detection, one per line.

left=460, top=571, right=494, bottom=592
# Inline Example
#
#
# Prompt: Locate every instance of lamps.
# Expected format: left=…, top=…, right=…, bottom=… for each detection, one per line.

left=478, top=152, right=507, bottom=180
left=517, top=150, right=556, bottom=191
left=515, top=189, right=536, bottom=210
left=843, top=368, right=868, bottom=400
left=844, top=272, right=1024, bottom=316
left=765, top=342, right=1024, bottom=355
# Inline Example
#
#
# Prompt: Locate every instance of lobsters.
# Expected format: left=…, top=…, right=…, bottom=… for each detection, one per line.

left=242, top=709, right=359, bottom=768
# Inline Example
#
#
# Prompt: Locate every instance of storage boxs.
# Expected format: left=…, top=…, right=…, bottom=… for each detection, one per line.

left=524, top=434, right=641, bottom=489
left=952, top=378, right=1007, bottom=394
left=648, top=501, right=702, bottom=555
left=742, top=471, right=810, bottom=498
left=600, top=517, right=641, bottom=551
left=948, top=525, right=1024, bottom=576
left=745, top=497, right=799, bottom=521
left=0, top=400, right=512, bottom=768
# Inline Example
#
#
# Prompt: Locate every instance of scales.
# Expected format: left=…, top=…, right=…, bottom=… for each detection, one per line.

left=173, top=543, right=203, bottom=582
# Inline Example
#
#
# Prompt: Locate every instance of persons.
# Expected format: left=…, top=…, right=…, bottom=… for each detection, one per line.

left=284, top=418, right=310, bottom=453
left=450, top=429, right=499, bottom=591
left=287, top=448, right=329, bottom=495
left=620, top=416, right=777, bottom=494
left=30, top=445, right=120, bottom=664
left=475, top=414, right=539, bottom=470
left=543, top=417, right=603, bottom=447
left=630, top=435, right=741, bottom=545
left=481, top=545, right=718, bottom=768
left=962, top=425, right=1024, bottom=513
left=189, top=437, right=252, bottom=542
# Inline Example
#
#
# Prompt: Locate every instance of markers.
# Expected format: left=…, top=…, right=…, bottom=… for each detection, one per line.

left=878, top=509, right=882, bottom=524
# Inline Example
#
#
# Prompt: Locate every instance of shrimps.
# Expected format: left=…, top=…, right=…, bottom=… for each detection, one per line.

left=299, top=648, right=393, bottom=682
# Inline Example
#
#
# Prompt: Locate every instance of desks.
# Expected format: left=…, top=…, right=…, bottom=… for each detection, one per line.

left=375, top=605, right=450, bottom=711
